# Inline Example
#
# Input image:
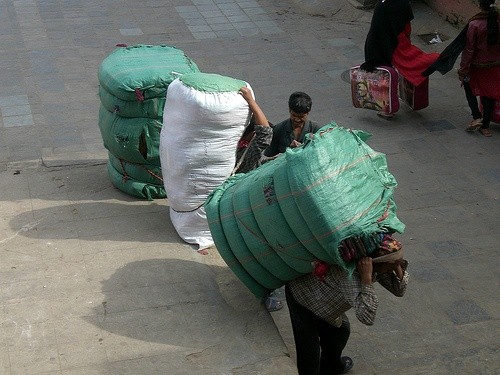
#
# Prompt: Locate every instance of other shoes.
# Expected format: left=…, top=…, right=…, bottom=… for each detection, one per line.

left=264, top=297, right=283, bottom=312
left=466, top=120, right=483, bottom=132
left=480, top=127, right=493, bottom=136
left=377, top=112, right=397, bottom=120
left=341, top=356, right=353, bottom=374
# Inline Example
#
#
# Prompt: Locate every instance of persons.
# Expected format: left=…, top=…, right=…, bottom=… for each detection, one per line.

left=457, top=0, right=500, bottom=137
left=262, top=91, right=324, bottom=165
left=235, top=87, right=276, bottom=179
left=364, top=0, right=416, bottom=119
left=284, top=256, right=409, bottom=375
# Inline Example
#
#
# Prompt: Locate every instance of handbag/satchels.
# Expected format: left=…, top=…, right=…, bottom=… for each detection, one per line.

left=400, top=74, right=429, bottom=111
left=350, top=65, right=399, bottom=113
